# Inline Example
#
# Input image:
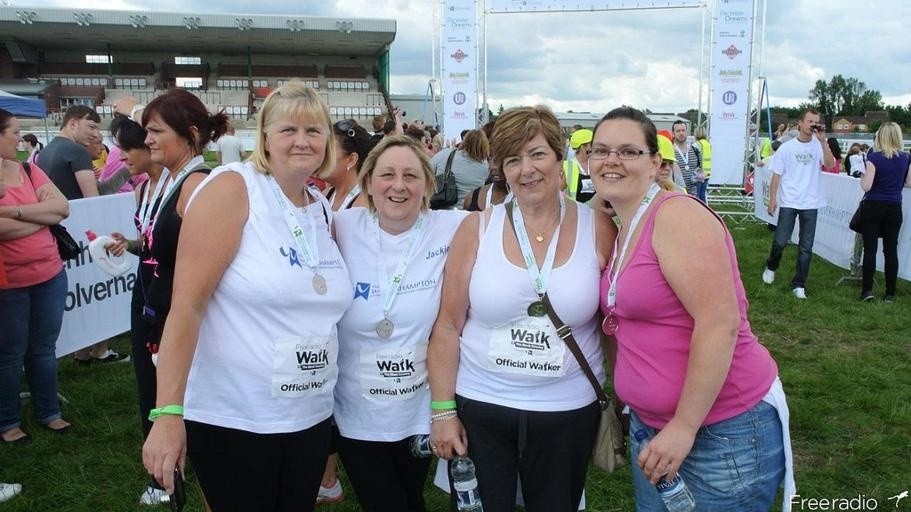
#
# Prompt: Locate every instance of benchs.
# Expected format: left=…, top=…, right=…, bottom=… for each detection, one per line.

left=1, top=60, right=392, bottom=141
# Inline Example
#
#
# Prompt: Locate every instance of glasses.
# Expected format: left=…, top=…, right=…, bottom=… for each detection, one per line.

left=586, top=147, right=653, bottom=160
left=334, top=119, right=357, bottom=137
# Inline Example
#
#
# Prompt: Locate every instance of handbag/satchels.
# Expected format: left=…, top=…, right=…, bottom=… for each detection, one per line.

left=849, top=199, right=866, bottom=233
left=592, top=393, right=631, bottom=475
left=430, top=174, right=458, bottom=208
left=50, top=224, right=81, bottom=260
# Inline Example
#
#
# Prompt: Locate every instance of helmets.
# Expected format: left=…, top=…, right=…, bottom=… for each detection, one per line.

left=656, top=133, right=678, bottom=163
left=570, top=129, right=593, bottom=149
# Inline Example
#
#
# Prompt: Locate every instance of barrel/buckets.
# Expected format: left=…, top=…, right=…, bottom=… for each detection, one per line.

left=84, top=229, right=133, bottom=278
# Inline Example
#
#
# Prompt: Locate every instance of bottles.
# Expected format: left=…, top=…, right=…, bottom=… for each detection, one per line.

left=410, top=433, right=432, bottom=457
left=450, top=455, right=485, bottom=512
left=634, top=428, right=697, bottom=510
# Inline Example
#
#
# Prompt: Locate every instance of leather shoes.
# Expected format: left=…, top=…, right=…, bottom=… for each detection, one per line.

left=47, top=418, right=72, bottom=433
left=2, top=426, right=32, bottom=446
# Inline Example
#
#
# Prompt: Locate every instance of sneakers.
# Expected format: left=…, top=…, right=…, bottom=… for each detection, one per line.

left=763, top=266, right=775, bottom=284
left=793, top=288, right=806, bottom=299
left=93, top=348, right=131, bottom=366
left=0, top=482, right=22, bottom=503
left=315, top=478, right=345, bottom=506
left=860, top=290, right=874, bottom=303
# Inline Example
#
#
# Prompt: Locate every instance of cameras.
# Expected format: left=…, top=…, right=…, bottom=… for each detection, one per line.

left=170, top=464, right=185, bottom=512
